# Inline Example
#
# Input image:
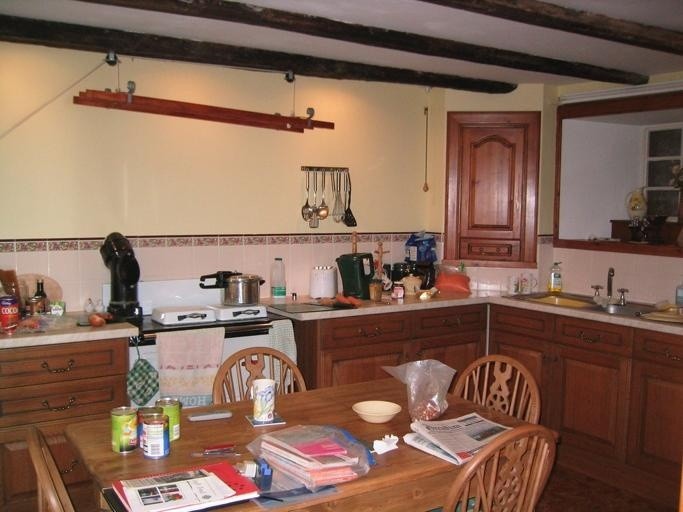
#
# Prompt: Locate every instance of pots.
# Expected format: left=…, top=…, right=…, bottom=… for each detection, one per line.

left=200, top=266, right=264, bottom=307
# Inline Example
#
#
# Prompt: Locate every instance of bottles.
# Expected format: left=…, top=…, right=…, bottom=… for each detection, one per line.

left=392, top=281, right=404, bottom=298
left=269, top=257, right=287, bottom=305
left=383, top=264, right=390, bottom=278
left=391, top=262, right=409, bottom=281
left=624, top=185, right=648, bottom=219
left=0, top=278, right=48, bottom=337
left=401, top=274, right=421, bottom=296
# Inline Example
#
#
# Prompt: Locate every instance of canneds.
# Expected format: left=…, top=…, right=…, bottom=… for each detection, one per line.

left=111, top=397, right=181, bottom=459
left=25, top=296, right=45, bottom=314
left=0, top=296, right=19, bottom=336
left=392, top=281, right=405, bottom=300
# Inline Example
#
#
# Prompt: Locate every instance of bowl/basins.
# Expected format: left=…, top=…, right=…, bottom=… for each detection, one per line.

left=351, top=400, right=401, bottom=424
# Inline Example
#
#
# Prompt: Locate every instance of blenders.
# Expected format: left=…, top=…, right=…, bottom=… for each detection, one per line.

left=99, top=232, right=143, bottom=323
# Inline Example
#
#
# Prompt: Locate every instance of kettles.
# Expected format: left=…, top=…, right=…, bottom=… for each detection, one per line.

left=335, top=252, right=374, bottom=297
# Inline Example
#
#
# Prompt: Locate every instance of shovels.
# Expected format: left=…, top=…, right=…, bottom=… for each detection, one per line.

left=309, top=170, right=320, bottom=228
left=342, top=172, right=357, bottom=227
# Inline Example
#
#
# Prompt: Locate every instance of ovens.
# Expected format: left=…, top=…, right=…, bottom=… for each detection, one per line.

left=127, top=330, right=299, bottom=410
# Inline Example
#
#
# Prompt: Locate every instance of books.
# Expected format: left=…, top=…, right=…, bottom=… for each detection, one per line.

left=103, top=431, right=359, bottom=512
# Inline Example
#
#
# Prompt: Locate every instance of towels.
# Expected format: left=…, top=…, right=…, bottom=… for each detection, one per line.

left=266, top=317, right=298, bottom=389
left=153, top=326, right=225, bottom=400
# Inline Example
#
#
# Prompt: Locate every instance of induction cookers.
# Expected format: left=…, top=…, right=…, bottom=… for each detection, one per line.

left=127, top=305, right=296, bottom=333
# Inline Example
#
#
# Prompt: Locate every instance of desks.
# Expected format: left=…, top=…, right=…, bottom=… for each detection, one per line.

left=63, top=374, right=530, bottom=511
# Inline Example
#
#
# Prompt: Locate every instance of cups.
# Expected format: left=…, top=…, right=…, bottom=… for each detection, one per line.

left=369, top=282, right=382, bottom=301
left=676, top=285, right=682, bottom=304
left=310, top=265, right=337, bottom=299
left=520, top=272, right=536, bottom=294
left=252, top=378, right=277, bottom=421
left=506, top=275, right=518, bottom=294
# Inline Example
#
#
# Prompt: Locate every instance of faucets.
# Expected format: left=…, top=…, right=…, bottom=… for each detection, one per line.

left=606, top=267, right=615, bottom=299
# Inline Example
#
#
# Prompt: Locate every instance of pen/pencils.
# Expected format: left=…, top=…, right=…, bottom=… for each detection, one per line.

left=190, top=444, right=245, bottom=456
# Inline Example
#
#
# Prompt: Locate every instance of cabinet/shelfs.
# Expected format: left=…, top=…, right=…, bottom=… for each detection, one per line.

left=440, top=108, right=542, bottom=270
left=550, top=304, right=626, bottom=493
left=0, top=327, right=143, bottom=499
left=406, top=297, right=489, bottom=398
left=551, top=85, right=682, bottom=256
left=265, top=307, right=408, bottom=391
left=625, top=320, right=680, bottom=512
left=484, top=295, right=550, bottom=432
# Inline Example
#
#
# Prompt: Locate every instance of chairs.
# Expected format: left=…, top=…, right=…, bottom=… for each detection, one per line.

left=24, top=416, right=77, bottom=512
left=442, top=420, right=558, bottom=512
left=455, top=353, right=544, bottom=425
left=210, top=345, right=307, bottom=406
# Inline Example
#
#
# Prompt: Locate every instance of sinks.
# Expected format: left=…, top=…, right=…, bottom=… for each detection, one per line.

left=502, top=291, right=600, bottom=310
